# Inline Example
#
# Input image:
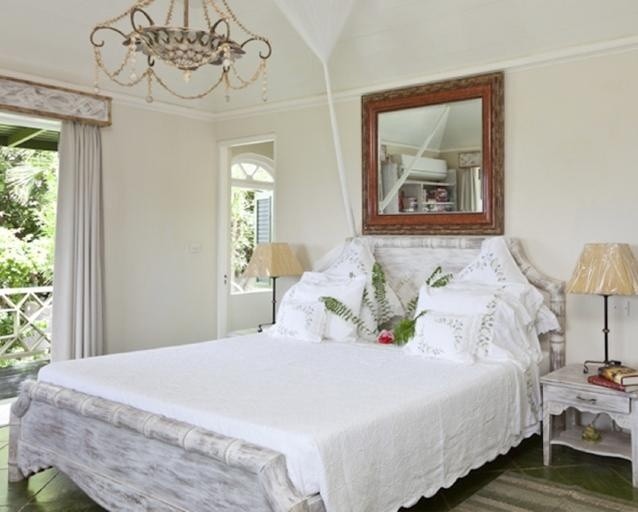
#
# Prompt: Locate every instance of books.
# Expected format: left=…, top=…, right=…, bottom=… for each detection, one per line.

left=588, top=365, right=638, bottom=393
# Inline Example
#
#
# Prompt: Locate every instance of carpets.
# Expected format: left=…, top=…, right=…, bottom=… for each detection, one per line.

left=449, top=471, right=638, bottom=511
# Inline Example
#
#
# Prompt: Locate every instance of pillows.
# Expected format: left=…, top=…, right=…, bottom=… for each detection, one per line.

left=274, top=235, right=556, bottom=371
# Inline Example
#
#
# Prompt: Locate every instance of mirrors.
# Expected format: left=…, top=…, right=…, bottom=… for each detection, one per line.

left=357, top=72, right=506, bottom=233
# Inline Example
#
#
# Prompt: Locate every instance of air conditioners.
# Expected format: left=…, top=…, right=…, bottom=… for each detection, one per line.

left=389, top=152, right=447, bottom=181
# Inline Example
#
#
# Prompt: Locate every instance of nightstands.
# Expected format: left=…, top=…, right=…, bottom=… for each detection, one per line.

left=537, top=362, right=638, bottom=494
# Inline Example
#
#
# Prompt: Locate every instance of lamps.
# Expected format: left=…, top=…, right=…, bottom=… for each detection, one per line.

left=89, top=0, right=273, bottom=109
left=565, top=241, right=638, bottom=376
left=241, top=240, right=306, bottom=336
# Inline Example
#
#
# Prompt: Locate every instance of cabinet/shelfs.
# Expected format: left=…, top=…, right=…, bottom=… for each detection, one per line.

left=383, top=165, right=458, bottom=213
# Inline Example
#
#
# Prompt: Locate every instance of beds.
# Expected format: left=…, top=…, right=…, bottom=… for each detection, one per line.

left=7, top=234, right=566, bottom=511
